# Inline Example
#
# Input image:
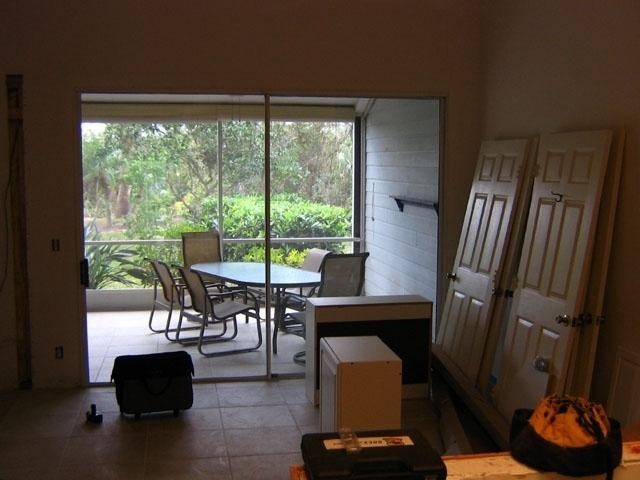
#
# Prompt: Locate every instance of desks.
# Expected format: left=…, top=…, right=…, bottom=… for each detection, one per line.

left=190, top=261, right=322, bottom=353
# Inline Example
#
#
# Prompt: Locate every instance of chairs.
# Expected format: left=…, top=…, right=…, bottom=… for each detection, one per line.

left=247, top=246, right=370, bottom=365
left=143, top=230, right=263, bottom=358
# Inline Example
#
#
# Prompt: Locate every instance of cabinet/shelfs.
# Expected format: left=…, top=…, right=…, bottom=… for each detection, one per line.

left=305, top=295, right=435, bottom=435
